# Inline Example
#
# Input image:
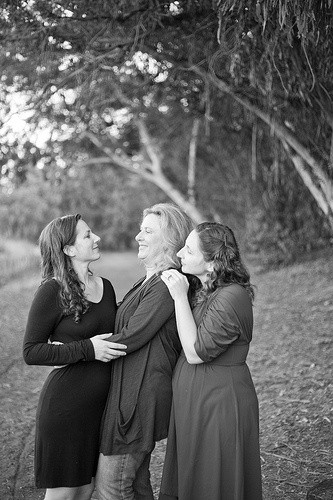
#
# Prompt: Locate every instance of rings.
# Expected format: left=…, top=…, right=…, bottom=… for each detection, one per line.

left=166, top=275, right=171, bottom=280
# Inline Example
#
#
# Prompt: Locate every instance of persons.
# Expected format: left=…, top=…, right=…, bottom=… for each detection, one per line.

left=159, top=223, right=262, bottom=500
left=22, top=213, right=127, bottom=500
left=52, top=203, right=193, bottom=500
